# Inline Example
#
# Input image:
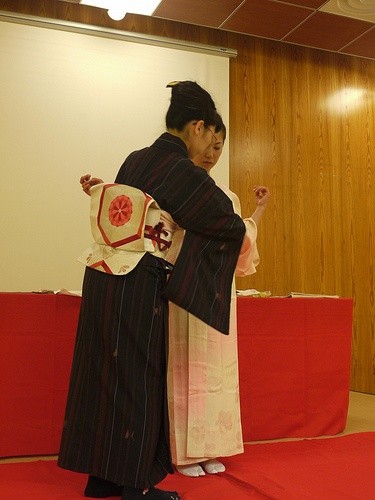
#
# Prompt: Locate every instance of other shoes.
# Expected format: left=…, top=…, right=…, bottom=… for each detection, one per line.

left=83, top=479, right=179, bottom=500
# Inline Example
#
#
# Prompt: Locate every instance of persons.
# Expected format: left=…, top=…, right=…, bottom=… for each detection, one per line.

left=58, top=78, right=251, bottom=500
left=79, top=111, right=270, bottom=477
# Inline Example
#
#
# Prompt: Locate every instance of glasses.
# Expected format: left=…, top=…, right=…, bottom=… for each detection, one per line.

left=192, top=122, right=217, bottom=143
